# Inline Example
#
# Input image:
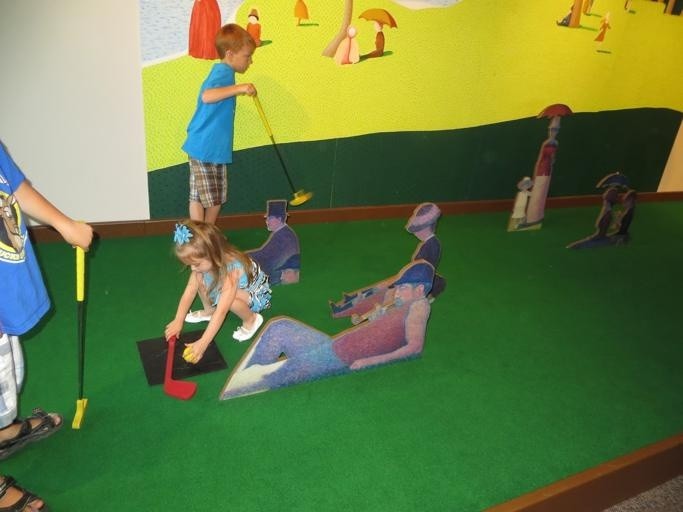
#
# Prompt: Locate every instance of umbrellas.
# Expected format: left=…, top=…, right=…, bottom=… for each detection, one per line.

left=596, top=172, right=631, bottom=188
left=536, top=104, right=573, bottom=119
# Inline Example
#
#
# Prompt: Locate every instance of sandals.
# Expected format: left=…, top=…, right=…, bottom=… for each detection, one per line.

left=0, top=476, right=47, bottom=512
left=0, top=412, right=62, bottom=460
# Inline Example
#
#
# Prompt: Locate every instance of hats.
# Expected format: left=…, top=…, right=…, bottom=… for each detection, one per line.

left=404, top=202, right=444, bottom=236
left=548, top=115, right=563, bottom=132
left=517, top=176, right=535, bottom=190
left=261, top=199, right=292, bottom=219
left=601, top=188, right=620, bottom=204
left=388, top=256, right=439, bottom=287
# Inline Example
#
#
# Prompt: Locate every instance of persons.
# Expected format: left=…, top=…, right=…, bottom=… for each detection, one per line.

left=165, top=219, right=272, bottom=364
left=0, top=140, right=93, bottom=512
left=508, top=177, right=532, bottom=231
left=526, top=117, right=561, bottom=224
left=610, top=190, right=635, bottom=234
left=181, top=24, right=257, bottom=224
left=566, top=185, right=620, bottom=249
left=220, top=258, right=435, bottom=400
left=243, top=214, right=301, bottom=287
left=329, top=202, right=440, bottom=317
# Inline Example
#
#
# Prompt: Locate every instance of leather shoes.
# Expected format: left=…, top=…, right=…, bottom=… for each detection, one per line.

left=232, top=313, right=264, bottom=341
left=185, top=310, right=212, bottom=324
